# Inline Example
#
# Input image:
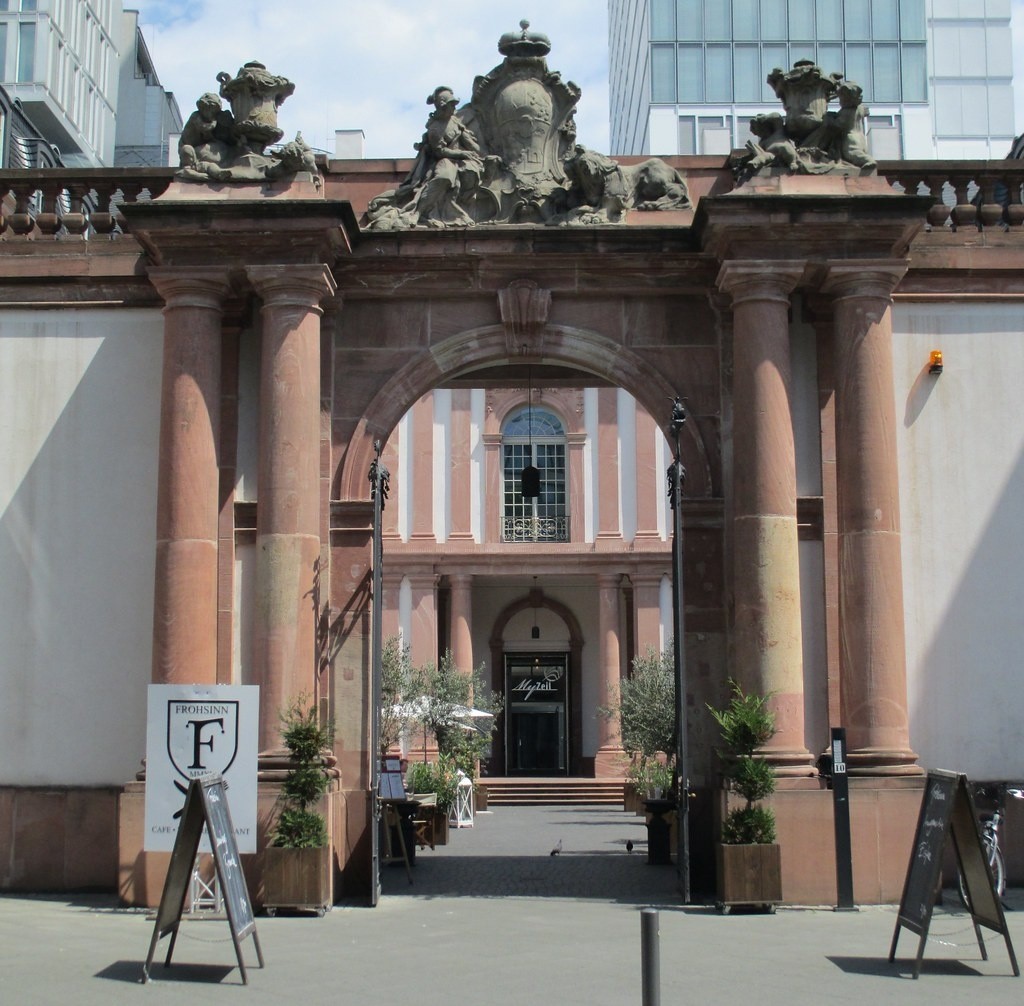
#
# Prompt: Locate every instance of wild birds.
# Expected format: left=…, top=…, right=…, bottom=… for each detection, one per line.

left=626, top=840, right=633, bottom=854
left=550, top=838, right=562, bottom=856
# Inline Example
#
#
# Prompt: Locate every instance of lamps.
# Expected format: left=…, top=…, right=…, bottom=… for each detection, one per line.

left=532, top=609, right=540, bottom=639
left=521, top=365, right=540, bottom=498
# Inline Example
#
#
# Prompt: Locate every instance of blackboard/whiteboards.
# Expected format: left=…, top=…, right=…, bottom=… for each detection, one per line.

left=896, top=767, right=1008, bottom=938
left=153, top=774, right=257, bottom=943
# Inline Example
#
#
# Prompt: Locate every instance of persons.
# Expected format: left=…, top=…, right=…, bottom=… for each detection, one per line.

left=410, top=87, right=481, bottom=224
left=178, top=93, right=318, bottom=181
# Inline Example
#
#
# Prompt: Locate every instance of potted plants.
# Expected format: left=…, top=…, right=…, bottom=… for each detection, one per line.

left=406, top=763, right=454, bottom=845
left=590, top=633, right=681, bottom=866
left=263, top=689, right=336, bottom=917
left=705, top=676, right=784, bottom=915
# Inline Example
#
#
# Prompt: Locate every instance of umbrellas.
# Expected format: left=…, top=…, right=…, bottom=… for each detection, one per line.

left=381, top=694, right=495, bottom=768
